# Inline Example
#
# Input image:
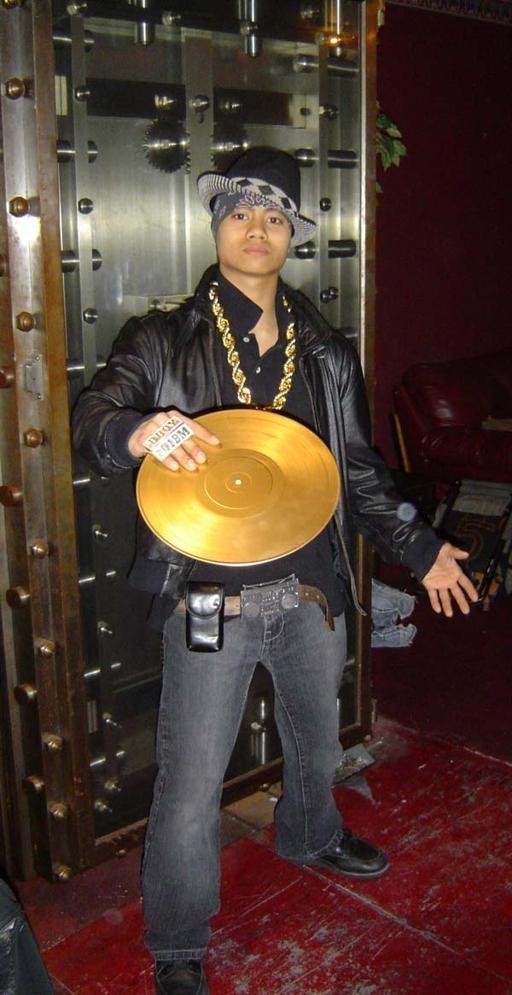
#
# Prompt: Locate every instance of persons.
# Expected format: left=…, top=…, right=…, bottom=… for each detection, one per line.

left=67, top=144, right=488, bottom=992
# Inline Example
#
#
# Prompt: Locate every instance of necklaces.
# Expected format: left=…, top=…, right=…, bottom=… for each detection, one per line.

left=203, top=280, right=301, bottom=412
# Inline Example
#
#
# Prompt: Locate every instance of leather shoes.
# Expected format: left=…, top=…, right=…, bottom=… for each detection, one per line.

left=313, top=830, right=390, bottom=879
left=153, top=960, right=210, bottom=995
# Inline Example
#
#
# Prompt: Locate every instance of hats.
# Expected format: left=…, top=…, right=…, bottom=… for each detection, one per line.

left=212, top=192, right=281, bottom=236
left=195, top=146, right=318, bottom=247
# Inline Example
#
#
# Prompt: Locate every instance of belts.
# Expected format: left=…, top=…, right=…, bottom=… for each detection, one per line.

left=172, top=584, right=335, bottom=632
